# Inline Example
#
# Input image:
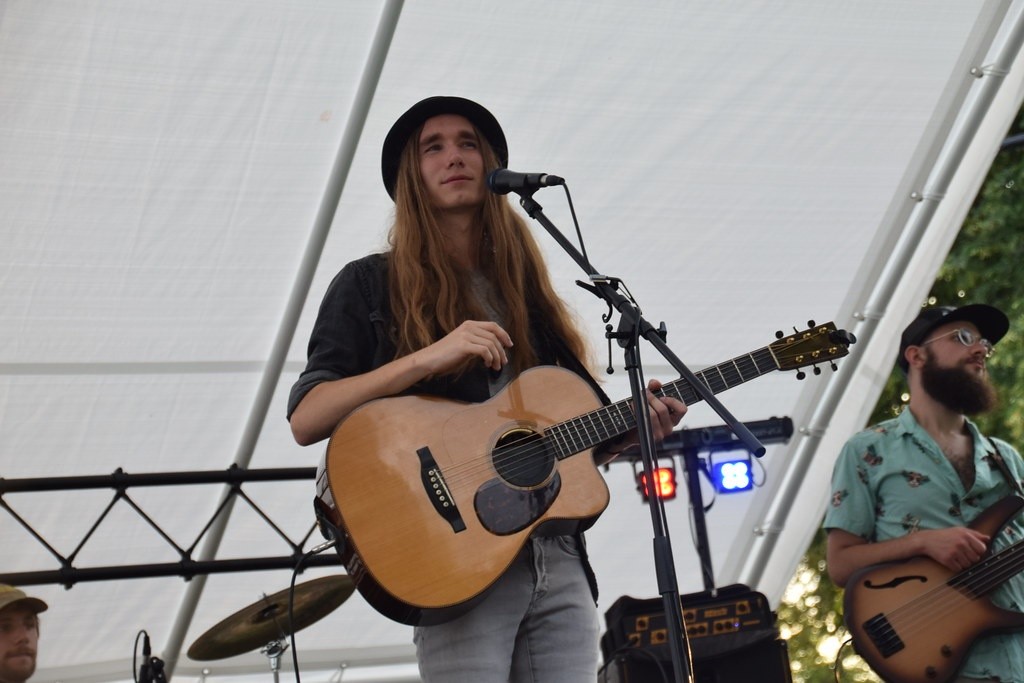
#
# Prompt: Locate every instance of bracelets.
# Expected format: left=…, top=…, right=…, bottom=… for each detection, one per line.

left=605, top=450, right=620, bottom=454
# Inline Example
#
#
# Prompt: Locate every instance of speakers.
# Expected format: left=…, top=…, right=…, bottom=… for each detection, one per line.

left=597, top=638, right=794, bottom=683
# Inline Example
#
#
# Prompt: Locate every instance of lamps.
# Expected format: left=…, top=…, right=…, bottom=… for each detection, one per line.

left=710, top=445, right=755, bottom=495
left=630, top=457, right=677, bottom=504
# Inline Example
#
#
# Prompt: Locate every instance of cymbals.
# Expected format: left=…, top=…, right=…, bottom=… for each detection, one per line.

left=187, top=572, right=356, bottom=662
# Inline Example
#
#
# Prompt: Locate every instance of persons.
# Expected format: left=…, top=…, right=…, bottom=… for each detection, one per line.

left=287, top=96, right=690, bottom=683
left=0, top=584, right=48, bottom=683
left=822, top=304, right=1024, bottom=683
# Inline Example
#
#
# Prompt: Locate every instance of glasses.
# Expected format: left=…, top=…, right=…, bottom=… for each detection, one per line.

left=921, top=328, right=995, bottom=358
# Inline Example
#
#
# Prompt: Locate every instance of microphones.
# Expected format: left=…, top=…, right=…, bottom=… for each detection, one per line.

left=488, top=167, right=565, bottom=196
left=137, top=634, right=153, bottom=683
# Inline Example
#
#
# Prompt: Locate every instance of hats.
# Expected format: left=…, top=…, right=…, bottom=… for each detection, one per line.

left=898, top=303, right=1009, bottom=375
left=0, top=583, right=48, bottom=613
left=381, top=96, right=508, bottom=203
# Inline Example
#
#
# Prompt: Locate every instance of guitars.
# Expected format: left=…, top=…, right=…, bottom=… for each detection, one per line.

left=312, top=316, right=861, bottom=630
left=841, top=489, right=1024, bottom=682
